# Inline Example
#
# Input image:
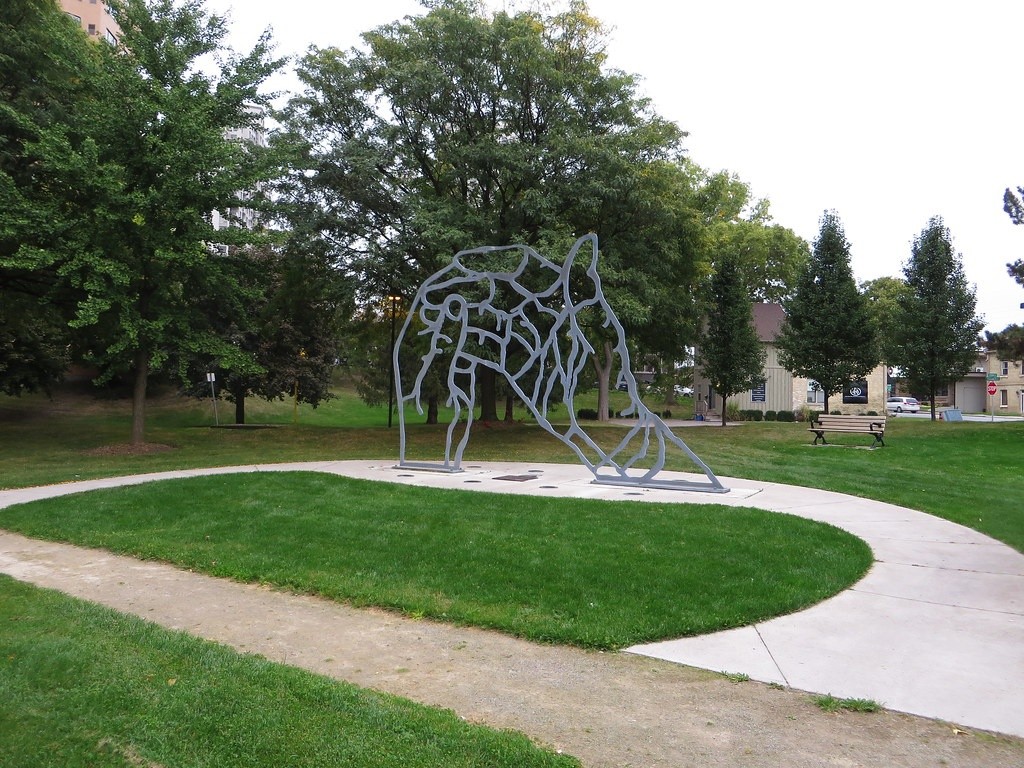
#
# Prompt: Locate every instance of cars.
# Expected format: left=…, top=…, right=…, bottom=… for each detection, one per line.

left=617, top=381, right=629, bottom=392
left=645, top=385, right=662, bottom=395
left=673, top=384, right=695, bottom=399
left=886, top=395, right=921, bottom=414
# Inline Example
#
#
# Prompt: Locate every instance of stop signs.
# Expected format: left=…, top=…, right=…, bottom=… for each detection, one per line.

left=988, top=382, right=998, bottom=395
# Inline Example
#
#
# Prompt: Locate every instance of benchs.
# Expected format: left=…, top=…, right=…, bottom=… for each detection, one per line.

left=806, top=413, right=887, bottom=451
